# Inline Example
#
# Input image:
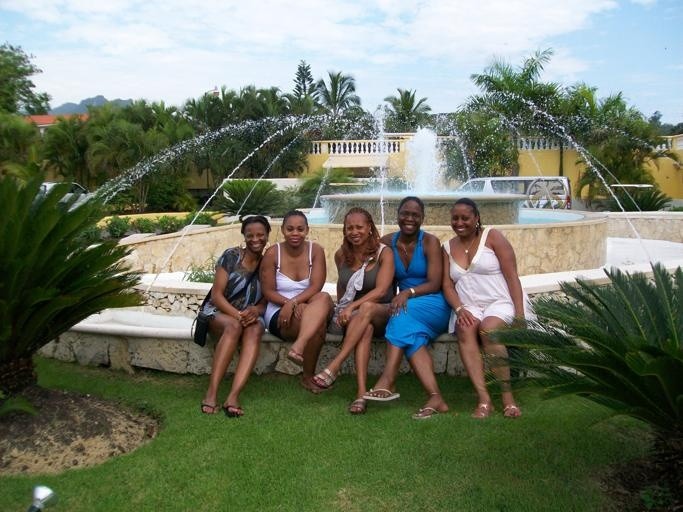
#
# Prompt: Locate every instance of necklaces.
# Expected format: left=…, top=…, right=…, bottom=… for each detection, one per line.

left=396, top=237, right=415, bottom=260
left=459, top=233, right=475, bottom=255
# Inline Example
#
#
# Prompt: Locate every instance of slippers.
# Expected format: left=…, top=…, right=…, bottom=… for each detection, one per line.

left=222, top=402, right=244, bottom=418
left=286, top=347, right=305, bottom=368
left=412, top=407, right=451, bottom=420
left=201, top=399, right=220, bottom=415
left=299, top=374, right=330, bottom=393
left=361, top=388, right=401, bottom=402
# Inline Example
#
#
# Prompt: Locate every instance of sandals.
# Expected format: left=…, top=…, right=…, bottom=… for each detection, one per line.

left=502, top=404, right=522, bottom=418
left=469, top=400, right=496, bottom=418
left=310, top=368, right=337, bottom=389
left=348, top=395, right=368, bottom=416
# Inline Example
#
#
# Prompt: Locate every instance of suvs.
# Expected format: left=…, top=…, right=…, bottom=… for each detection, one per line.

left=35, top=181, right=96, bottom=211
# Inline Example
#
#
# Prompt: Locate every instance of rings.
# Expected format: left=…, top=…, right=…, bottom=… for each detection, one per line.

left=341, top=319, right=346, bottom=325
left=282, top=318, right=286, bottom=323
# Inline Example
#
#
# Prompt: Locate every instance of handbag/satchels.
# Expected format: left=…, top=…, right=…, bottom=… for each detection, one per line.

left=193, top=312, right=210, bottom=348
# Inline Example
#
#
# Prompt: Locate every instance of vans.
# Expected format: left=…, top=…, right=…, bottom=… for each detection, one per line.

left=454, top=176, right=572, bottom=209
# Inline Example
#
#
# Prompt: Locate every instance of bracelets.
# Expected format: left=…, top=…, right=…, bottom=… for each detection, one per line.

left=237, top=310, right=242, bottom=321
left=409, top=286, right=416, bottom=298
left=290, top=295, right=297, bottom=307
left=454, top=305, right=464, bottom=313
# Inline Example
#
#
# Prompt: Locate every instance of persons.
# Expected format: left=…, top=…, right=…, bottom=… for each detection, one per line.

left=440, top=197, right=537, bottom=419
left=257, top=210, right=333, bottom=394
left=361, top=196, right=451, bottom=420
left=310, top=208, right=397, bottom=414
left=199, top=217, right=269, bottom=418
